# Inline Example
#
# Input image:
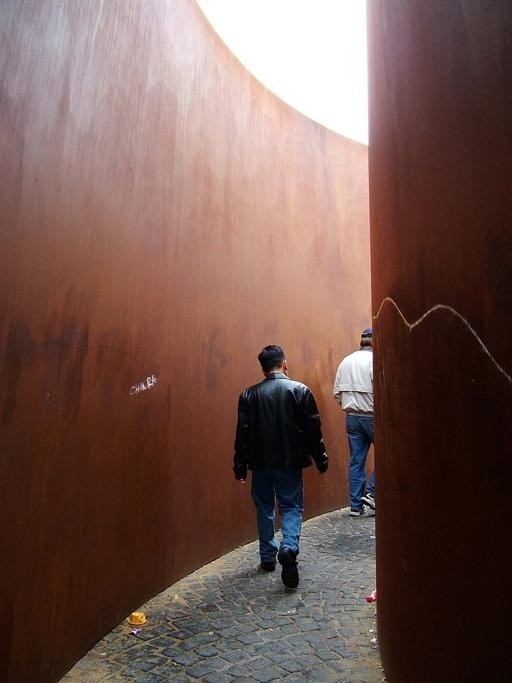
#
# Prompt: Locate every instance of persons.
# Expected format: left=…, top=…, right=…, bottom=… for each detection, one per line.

left=232, top=343, right=330, bottom=589
left=332, top=327, right=377, bottom=517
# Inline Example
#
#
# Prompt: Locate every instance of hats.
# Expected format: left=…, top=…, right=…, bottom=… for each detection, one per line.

left=359, top=326, right=372, bottom=337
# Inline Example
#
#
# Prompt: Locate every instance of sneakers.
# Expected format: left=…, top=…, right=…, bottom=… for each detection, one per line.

left=278, top=550, right=300, bottom=586
left=360, top=492, right=377, bottom=510
left=350, top=504, right=366, bottom=517
left=260, top=561, right=277, bottom=573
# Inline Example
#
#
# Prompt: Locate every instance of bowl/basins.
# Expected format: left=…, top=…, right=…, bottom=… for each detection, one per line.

left=128, top=612, right=148, bottom=625
